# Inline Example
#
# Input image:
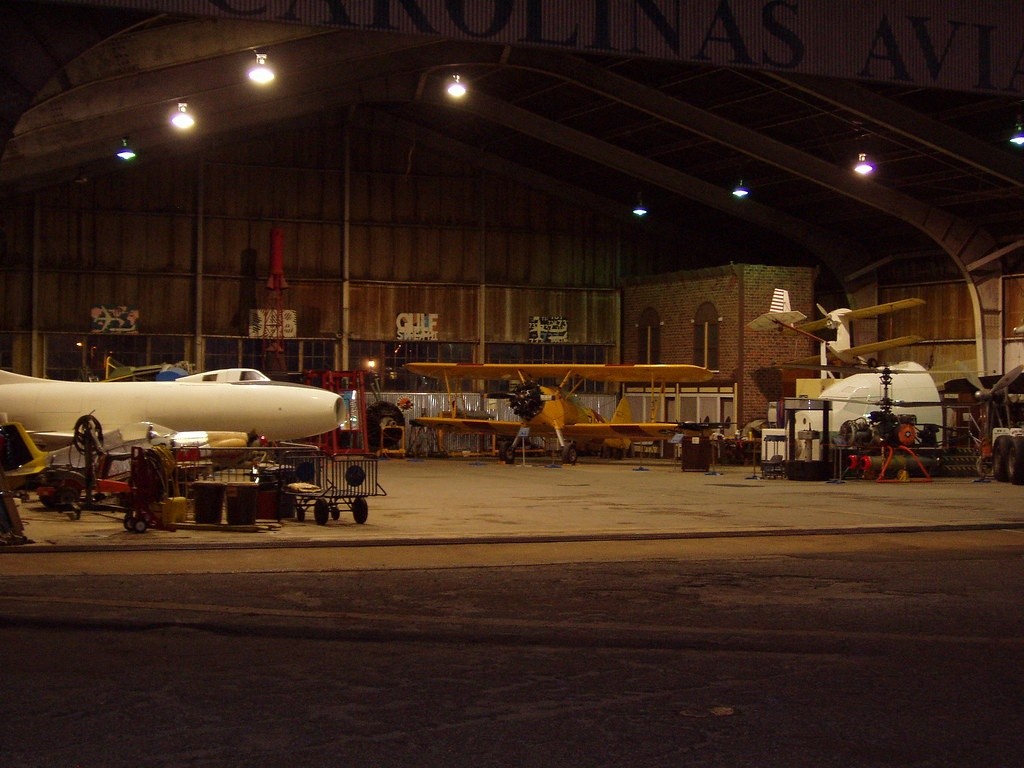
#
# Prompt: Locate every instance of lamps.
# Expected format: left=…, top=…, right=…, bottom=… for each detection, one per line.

left=117, top=140, right=136, bottom=159
left=250, top=55, right=275, bottom=84
left=75, top=167, right=88, bottom=183
left=171, top=104, right=194, bottom=129
left=731, top=182, right=749, bottom=196
left=632, top=200, right=648, bottom=214
left=447, top=75, right=467, bottom=97
left=853, top=155, right=873, bottom=173
left=1009, top=125, right=1024, bottom=145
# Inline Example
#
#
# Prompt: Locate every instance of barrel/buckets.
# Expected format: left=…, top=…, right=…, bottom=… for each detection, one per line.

left=225, top=483, right=258, bottom=525
left=257, top=491, right=277, bottom=519
left=186, top=482, right=226, bottom=524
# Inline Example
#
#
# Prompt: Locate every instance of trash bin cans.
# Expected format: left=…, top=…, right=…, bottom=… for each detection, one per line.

left=682, top=436, right=712, bottom=472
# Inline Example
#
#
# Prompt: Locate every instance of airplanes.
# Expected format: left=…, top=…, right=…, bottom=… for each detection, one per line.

left=405, top=362, right=715, bottom=465
left=0, top=413, right=249, bottom=532
left=0, top=363, right=352, bottom=511
left=747, top=297, right=925, bottom=370
